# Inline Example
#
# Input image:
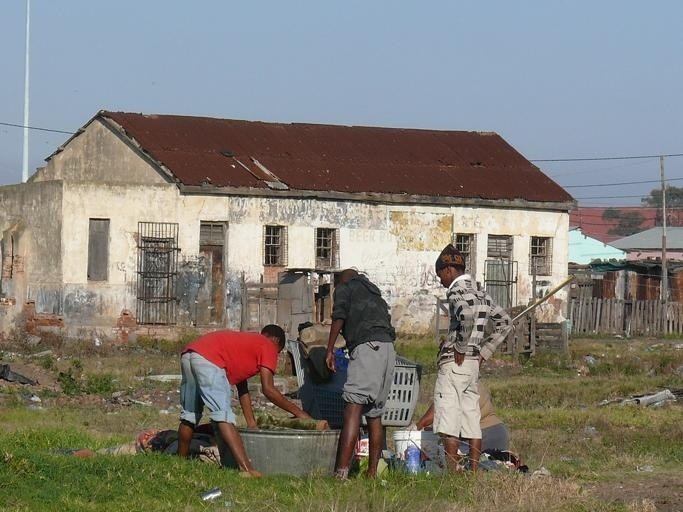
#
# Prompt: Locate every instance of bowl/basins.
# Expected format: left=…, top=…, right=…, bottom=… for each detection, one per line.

left=214, top=426, right=344, bottom=477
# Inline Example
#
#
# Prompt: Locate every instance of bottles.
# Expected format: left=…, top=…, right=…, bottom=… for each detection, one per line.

left=405, top=444, right=420, bottom=475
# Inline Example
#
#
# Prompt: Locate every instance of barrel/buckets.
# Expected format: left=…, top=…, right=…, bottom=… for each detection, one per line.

left=393, top=430, right=440, bottom=474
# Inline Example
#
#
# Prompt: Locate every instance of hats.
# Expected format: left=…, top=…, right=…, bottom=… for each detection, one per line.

left=435, top=243, right=466, bottom=272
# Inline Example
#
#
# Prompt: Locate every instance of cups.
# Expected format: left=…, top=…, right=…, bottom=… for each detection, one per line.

left=200, top=487, right=222, bottom=501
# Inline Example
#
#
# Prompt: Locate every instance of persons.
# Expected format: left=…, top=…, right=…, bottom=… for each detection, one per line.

left=416, top=336, right=512, bottom=470
left=429, top=243, right=514, bottom=475
left=325, top=267, right=398, bottom=483
left=176, top=322, right=315, bottom=479
